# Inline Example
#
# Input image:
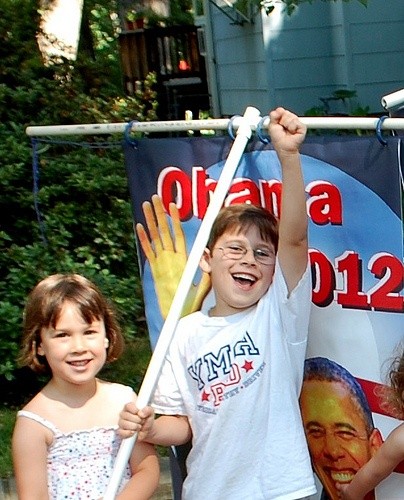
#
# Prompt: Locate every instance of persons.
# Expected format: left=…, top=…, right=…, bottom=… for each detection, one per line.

left=135, top=193, right=386, bottom=499
left=11, top=274, right=162, bottom=500
left=116, top=105, right=316, bottom=499
left=336, top=351, right=403, bottom=499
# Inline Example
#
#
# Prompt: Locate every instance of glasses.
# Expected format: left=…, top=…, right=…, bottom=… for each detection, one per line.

left=209, top=245, right=276, bottom=266
